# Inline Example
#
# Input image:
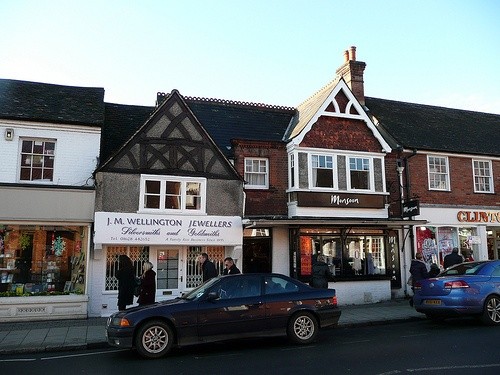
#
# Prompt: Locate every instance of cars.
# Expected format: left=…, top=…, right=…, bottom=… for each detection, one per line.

left=413, top=261, right=500, bottom=325
left=106, top=275, right=338, bottom=357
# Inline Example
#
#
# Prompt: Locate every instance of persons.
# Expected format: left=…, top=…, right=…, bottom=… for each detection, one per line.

left=222, top=257, right=241, bottom=275
left=312, top=253, right=336, bottom=288
left=136, top=261, right=156, bottom=306
left=198, top=253, right=218, bottom=283
left=428, top=263, right=441, bottom=278
left=409, top=252, right=428, bottom=307
left=115, top=254, right=138, bottom=311
left=444, top=247, right=463, bottom=270
